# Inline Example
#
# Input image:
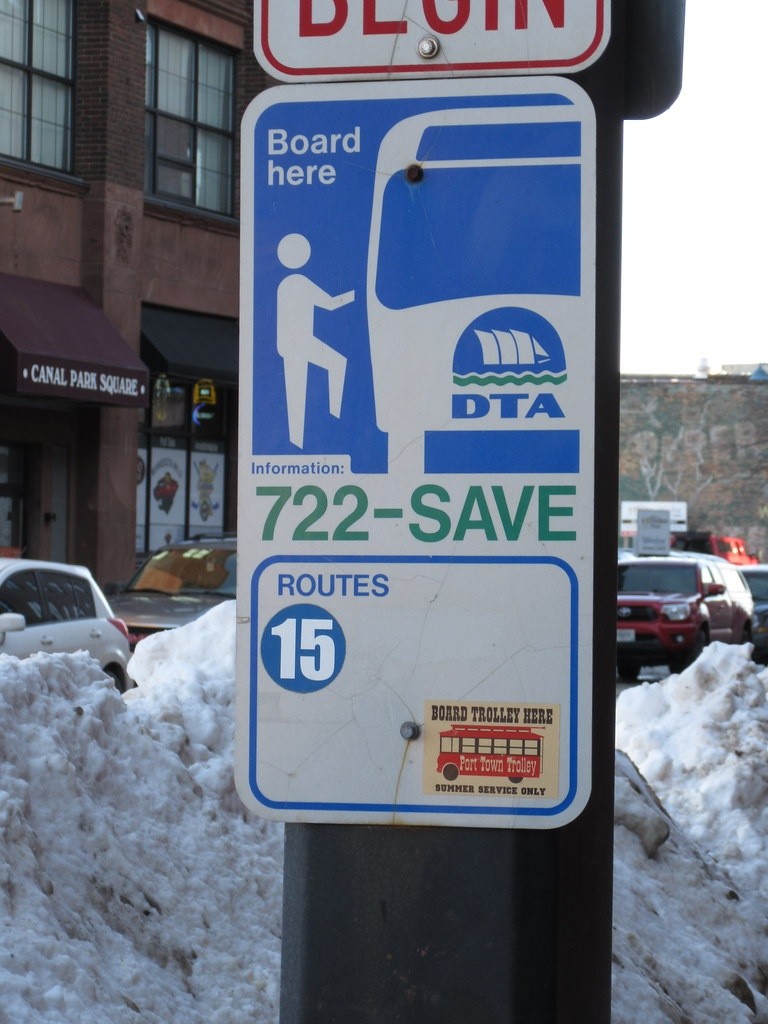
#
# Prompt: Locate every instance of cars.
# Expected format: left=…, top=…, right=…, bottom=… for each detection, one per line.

left=736, top=563, right=767, bottom=655
left=0, top=554, right=138, bottom=697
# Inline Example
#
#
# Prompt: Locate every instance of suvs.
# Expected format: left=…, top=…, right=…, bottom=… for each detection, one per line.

left=113, top=532, right=236, bottom=644
left=669, top=531, right=757, bottom=571
left=613, top=553, right=756, bottom=679
